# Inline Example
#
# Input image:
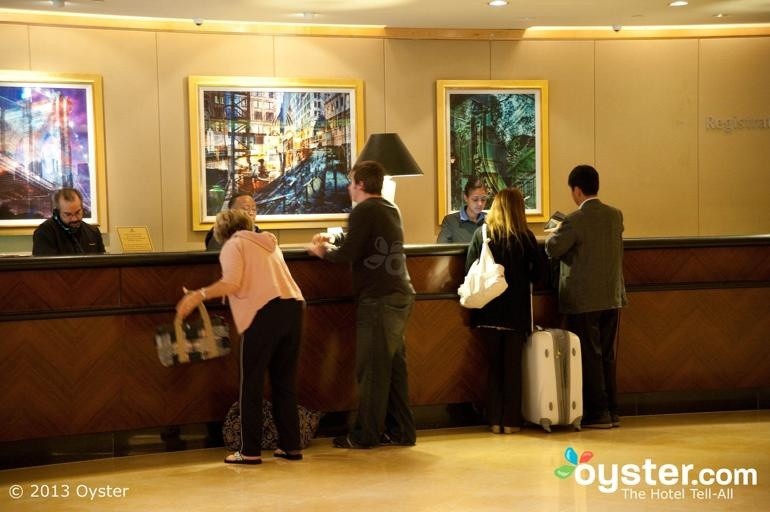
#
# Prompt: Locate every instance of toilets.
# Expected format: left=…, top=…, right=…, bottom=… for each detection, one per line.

left=53, top=209, right=71, bottom=234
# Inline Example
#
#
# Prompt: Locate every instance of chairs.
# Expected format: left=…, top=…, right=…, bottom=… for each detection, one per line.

left=582, top=412, right=612, bottom=429
left=378, top=432, right=413, bottom=446
left=333, top=434, right=372, bottom=449
left=611, top=415, right=619, bottom=426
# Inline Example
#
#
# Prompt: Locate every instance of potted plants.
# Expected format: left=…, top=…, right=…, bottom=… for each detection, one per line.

left=436, top=78, right=550, bottom=223
left=188, top=75, right=364, bottom=232
left=0, top=68, right=109, bottom=236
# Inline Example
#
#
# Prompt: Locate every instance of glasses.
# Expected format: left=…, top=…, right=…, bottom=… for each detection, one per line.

left=351, top=133, right=425, bottom=204
left=667, top=0, right=689, bottom=7
left=487, top=1, right=509, bottom=7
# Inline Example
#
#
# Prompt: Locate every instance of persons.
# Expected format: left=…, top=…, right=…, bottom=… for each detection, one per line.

left=33, top=187, right=106, bottom=255
left=311, top=161, right=416, bottom=448
left=206, top=192, right=261, bottom=252
left=464, top=188, right=538, bottom=435
left=175, top=209, right=307, bottom=465
left=545, top=164, right=629, bottom=428
left=437, top=178, right=488, bottom=244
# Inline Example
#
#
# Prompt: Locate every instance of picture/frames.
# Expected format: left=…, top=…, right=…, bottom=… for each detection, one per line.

left=1, top=234, right=770, bottom=457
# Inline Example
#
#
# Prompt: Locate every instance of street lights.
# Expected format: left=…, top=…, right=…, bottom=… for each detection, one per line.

left=457, top=223, right=508, bottom=310
left=155, top=301, right=231, bottom=367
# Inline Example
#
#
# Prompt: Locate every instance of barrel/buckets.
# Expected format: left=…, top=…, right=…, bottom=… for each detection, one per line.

left=273, top=447, right=303, bottom=460
left=224, top=451, right=262, bottom=464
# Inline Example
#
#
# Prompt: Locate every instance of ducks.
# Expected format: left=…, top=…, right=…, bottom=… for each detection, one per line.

left=521, top=282, right=583, bottom=433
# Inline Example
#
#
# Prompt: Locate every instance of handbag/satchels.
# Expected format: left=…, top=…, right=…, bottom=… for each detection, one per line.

left=201, top=288, right=206, bottom=299
